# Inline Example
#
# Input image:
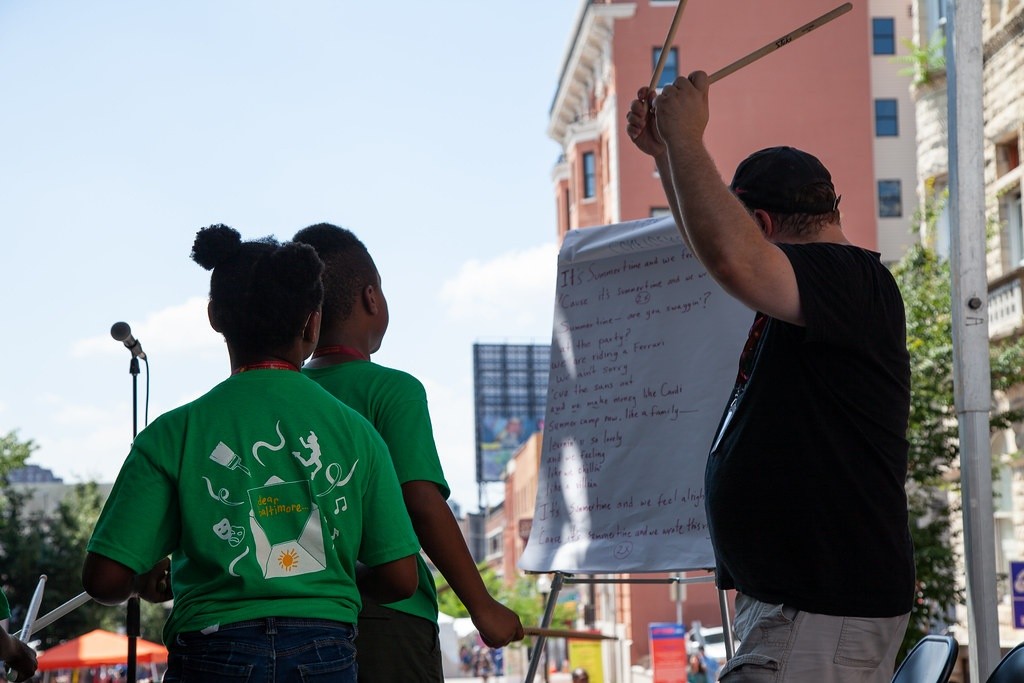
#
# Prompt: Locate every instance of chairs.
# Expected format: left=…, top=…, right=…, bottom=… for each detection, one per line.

left=985, top=642, right=1024, bottom=683
left=889, top=633, right=958, bottom=683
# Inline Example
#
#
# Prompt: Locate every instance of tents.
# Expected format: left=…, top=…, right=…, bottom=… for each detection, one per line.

left=36, top=627, right=169, bottom=683
left=437, top=610, right=478, bottom=679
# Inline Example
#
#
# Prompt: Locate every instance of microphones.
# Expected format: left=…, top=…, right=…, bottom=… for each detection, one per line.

left=110, top=321, right=147, bottom=361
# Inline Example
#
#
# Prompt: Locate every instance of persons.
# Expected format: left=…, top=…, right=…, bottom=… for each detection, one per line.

left=81, top=225, right=420, bottom=683
left=687, top=654, right=708, bottom=683
left=697, top=645, right=719, bottom=683
left=460, top=643, right=504, bottom=683
left=0, top=588, right=38, bottom=683
left=626, top=70, right=916, bottom=683
left=294, top=222, right=525, bottom=683
